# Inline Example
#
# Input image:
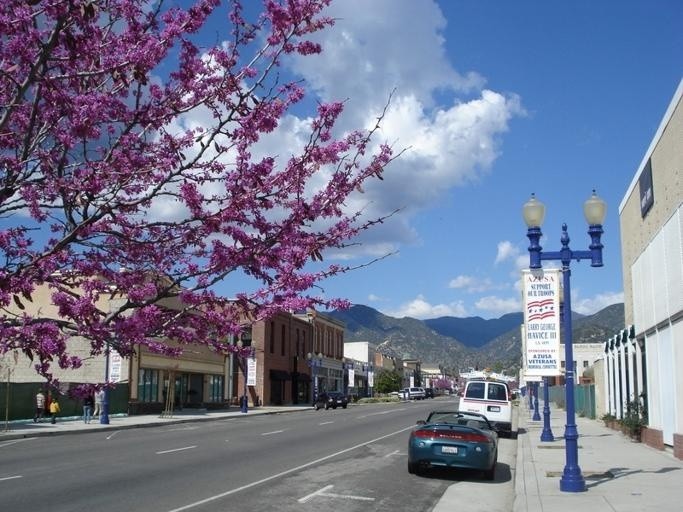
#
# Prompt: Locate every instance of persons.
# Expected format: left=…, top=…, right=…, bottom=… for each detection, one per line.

left=43, top=390, right=53, bottom=420
left=49, top=398, right=62, bottom=424
left=32, top=388, right=45, bottom=422
left=79, top=395, right=94, bottom=423
left=92, top=386, right=105, bottom=420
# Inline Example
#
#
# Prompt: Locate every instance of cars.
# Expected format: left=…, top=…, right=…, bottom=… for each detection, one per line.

left=391, top=390, right=409, bottom=400
left=313, top=391, right=348, bottom=410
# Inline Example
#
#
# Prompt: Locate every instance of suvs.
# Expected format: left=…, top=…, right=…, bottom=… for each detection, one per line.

left=423, top=388, right=434, bottom=399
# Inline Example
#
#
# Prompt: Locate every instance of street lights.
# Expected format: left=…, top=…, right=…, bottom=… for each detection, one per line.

left=341, top=357, right=355, bottom=397
left=237, top=340, right=257, bottom=408
left=522, top=189, right=606, bottom=465
left=307, top=352, right=323, bottom=402
left=362, top=360, right=373, bottom=394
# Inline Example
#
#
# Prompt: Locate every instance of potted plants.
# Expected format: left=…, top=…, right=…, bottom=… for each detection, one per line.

left=597, top=390, right=646, bottom=440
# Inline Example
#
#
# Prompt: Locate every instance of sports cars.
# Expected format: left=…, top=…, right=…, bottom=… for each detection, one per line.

left=408, top=410, right=503, bottom=480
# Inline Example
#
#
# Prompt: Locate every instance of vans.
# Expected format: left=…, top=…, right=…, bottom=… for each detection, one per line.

left=404, top=387, right=426, bottom=399
left=457, top=381, right=516, bottom=436
